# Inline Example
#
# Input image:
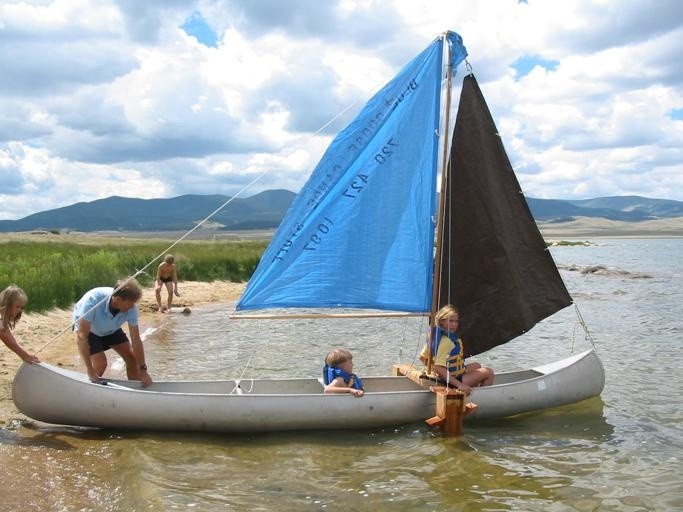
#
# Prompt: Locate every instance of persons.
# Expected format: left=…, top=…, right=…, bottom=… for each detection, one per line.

left=70, top=275, right=154, bottom=391
left=153, top=253, right=181, bottom=314
left=320, top=347, right=366, bottom=397
left=418, top=302, right=495, bottom=398
left=1, top=284, right=42, bottom=366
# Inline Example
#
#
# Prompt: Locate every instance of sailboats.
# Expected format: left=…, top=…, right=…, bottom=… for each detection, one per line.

left=10, top=28, right=609, bottom=435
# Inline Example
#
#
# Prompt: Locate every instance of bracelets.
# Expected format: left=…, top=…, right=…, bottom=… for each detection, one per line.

left=136, top=363, right=149, bottom=371
